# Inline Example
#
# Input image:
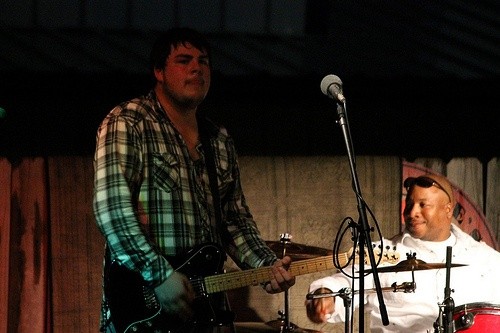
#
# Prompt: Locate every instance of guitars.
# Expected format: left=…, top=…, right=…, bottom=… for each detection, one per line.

left=105, top=243, right=400, bottom=333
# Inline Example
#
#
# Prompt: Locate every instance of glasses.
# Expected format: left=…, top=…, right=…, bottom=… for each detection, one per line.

left=403, top=177, right=451, bottom=204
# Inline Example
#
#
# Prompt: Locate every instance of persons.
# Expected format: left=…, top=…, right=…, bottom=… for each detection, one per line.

left=92, top=27, right=296, bottom=333
left=305, top=174, right=500, bottom=333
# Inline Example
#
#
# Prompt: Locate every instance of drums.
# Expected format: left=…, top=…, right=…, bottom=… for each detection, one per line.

left=433, top=302, right=500, bottom=333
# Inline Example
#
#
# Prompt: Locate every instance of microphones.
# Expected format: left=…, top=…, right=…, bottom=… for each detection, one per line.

left=320, top=74, right=346, bottom=103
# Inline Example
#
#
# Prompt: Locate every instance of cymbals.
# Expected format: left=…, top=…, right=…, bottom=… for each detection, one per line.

left=233, top=318, right=324, bottom=332
left=355, top=257, right=468, bottom=272
